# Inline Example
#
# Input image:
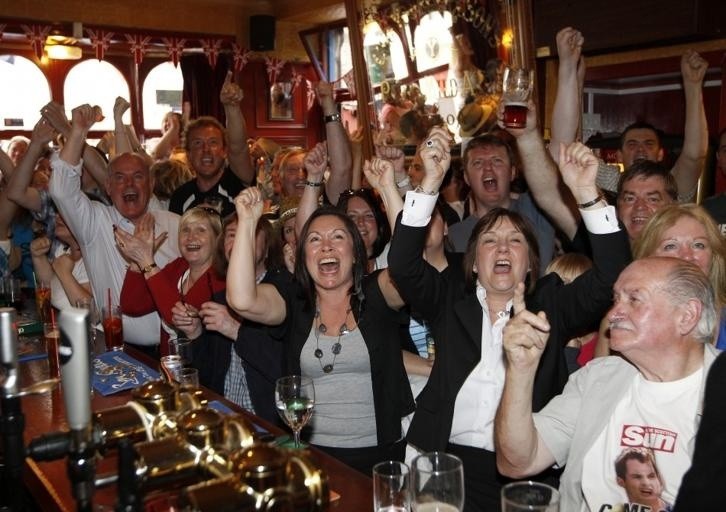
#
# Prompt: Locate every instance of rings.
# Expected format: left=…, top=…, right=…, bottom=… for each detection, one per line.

left=427, top=140, right=431, bottom=145
left=119, top=242, right=123, bottom=247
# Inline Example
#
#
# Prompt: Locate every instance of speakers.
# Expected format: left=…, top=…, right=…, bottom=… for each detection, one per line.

left=249, top=13, right=276, bottom=52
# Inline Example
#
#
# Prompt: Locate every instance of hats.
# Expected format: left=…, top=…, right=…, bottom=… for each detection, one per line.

left=457, top=102, right=493, bottom=135
left=274, top=198, right=302, bottom=223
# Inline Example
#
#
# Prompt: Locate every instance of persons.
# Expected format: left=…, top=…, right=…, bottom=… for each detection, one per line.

left=167, top=70, right=253, bottom=223
left=701, top=130, right=725, bottom=235
left=172, top=213, right=311, bottom=433
left=0, top=103, right=65, bottom=310
left=548, top=28, right=709, bottom=202
left=500, top=104, right=684, bottom=279
left=293, top=140, right=405, bottom=274
left=113, top=206, right=229, bottom=369
left=495, top=256, right=724, bottom=510
left=50, top=97, right=181, bottom=333
left=227, top=185, right=416, bottom=489
left=376, top=201, right=465, bottom=436
left=257, top=76, right=352, bottom=211
left=636, top=202, right=722, bottom=326
left=249, top=139, right=305, bottom=202
left=388, top=127, right=634, bottom=511
left=30, top=192, right=108, bottom=310
left=545, top=257, right=599, bottom=373
left=615, top=448, right=672, bottom=512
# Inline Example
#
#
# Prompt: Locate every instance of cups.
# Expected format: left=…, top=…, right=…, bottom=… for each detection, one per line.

left=5, top=279, right=21, bottom=314
left=169, top=338, right=193, bottom=368
left=503, top=67, right=534, bottom=129
left=372, top=461, right=410, bottom=512
left=410, top=452, right=464, bottom=511
left=77, top=296, right=97, bottom=344
left=276, top=376, right=315, bottom=449
left=34, top=273, right=52, bottom=323
left=502, top=482, right=560, bottom=512
left=101, top=305, right=124, bottom=353
left=174, top=368, right=198, bottom=410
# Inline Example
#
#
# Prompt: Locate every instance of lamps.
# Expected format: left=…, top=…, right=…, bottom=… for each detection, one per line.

left=43, top=36, right=81, bottom=60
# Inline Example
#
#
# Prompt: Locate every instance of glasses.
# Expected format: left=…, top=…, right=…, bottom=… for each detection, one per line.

left=196, top=204, right=223, bottom=217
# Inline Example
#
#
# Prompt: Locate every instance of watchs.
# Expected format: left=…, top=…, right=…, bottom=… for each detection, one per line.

left=324, top=113, right=340, bottom=123
left=141, top=263, right=158, bottom=275
left=397, top=176, right=411, bottom=189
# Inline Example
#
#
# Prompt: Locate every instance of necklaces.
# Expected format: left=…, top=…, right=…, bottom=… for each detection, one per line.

left=314, top=302, right=352, bottom=374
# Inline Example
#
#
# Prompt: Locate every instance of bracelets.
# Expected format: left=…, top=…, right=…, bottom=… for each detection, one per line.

left=578, top=196, right=602, bottom=210
left=134, top=146, right=143, bottom=151
left=304, top=180, right=325, bottom=188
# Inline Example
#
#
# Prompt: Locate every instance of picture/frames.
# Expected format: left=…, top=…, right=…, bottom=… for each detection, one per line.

left=256, top=135, right=309, bottom=153
left=268, top=82, right=294, bottom=121
